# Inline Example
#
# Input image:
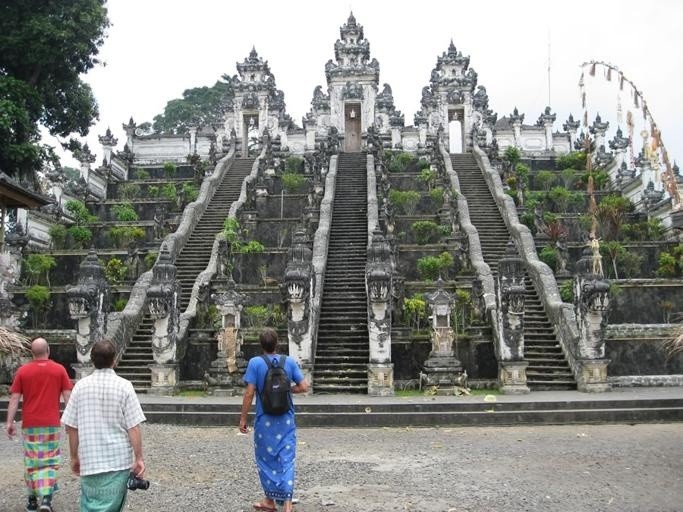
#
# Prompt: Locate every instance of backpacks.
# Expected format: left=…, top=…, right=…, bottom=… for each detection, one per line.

left=257, top=354, right=291, bottom=416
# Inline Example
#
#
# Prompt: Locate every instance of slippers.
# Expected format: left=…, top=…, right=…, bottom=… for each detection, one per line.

left=253, top=501, right=277, bottom=512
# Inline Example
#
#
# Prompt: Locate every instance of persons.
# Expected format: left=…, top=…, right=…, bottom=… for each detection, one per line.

left=6, top=337, right=74, bottom=512
left=239, top=330, right=309, bottom=512
left=60, top=341, right=147, bottom=512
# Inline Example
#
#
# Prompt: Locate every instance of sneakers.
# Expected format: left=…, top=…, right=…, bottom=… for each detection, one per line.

left=25, top=494, right=54, bottom=512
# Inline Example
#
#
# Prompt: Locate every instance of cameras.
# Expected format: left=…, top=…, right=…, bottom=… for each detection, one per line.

left=127, top=472, right=150, bottom=490
